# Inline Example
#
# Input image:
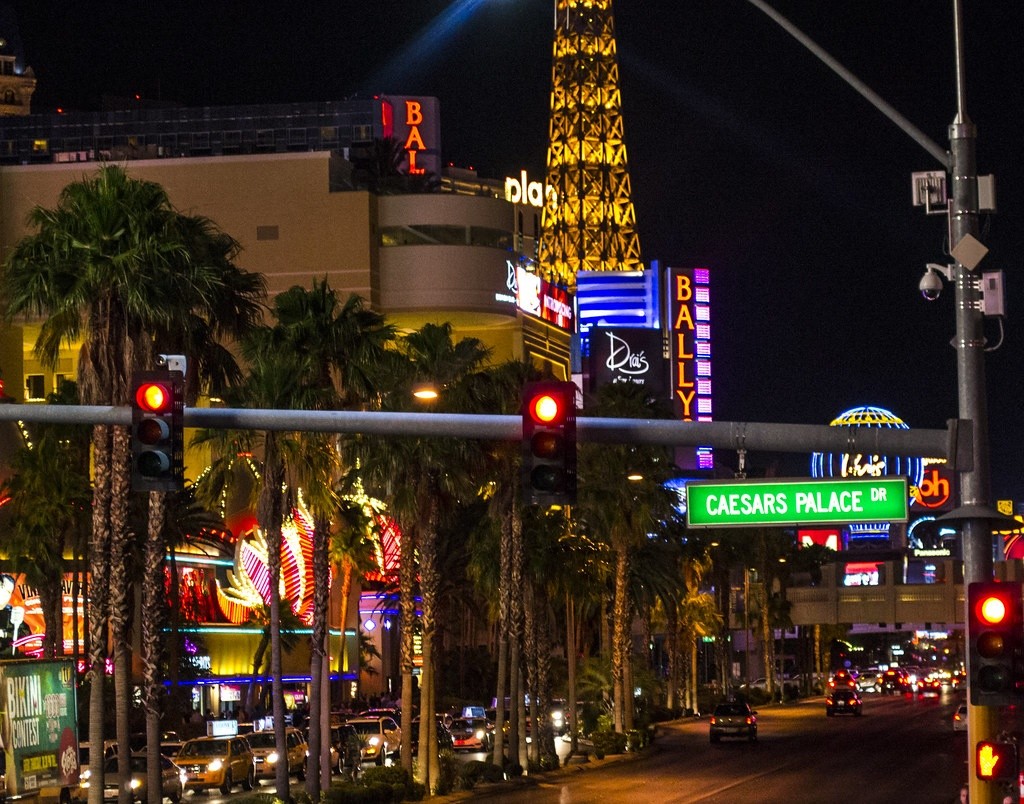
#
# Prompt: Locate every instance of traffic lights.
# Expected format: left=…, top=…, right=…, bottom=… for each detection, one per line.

left=524, top=378, right=581, bottom=516
left=131, top=369, right=188, bottom=496
left=967, top=579, right=1022, bottom=708
left=976, top=740, right=1017, bottom=783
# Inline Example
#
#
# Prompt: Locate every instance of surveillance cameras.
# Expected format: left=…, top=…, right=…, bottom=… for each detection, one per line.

left=919, top=272, right=942, bottom=301
left=156, top=354, right=166, bottom=365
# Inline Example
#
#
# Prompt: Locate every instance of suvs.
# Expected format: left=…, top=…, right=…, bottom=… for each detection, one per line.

left=345, top=716, right=403, bottom=769
left=246, top=727, right=309, bottom=781
left=174, top=734, right=257, bottom=797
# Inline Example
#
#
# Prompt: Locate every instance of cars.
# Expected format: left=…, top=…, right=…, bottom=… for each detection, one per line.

left=70, top=715, right=293, bottom=777
left=709, top=701, right=759, bottom=742
left=952, top=704, right=968, bottom=732
left=304, top=723, right=362, bottom=774
left=739, top=665, right=967, bottom=693
left=356, top=697, right=617, bottom=753
left=74, top=751, right=185, bottom=804
left=826, top=689, right=863, bottom=717
